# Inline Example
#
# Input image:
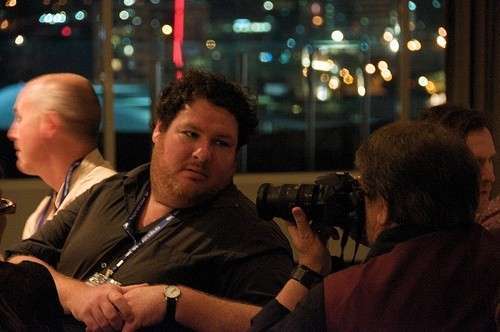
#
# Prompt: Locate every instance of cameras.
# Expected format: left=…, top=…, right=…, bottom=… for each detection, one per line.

left=257, top=172, right=365, bottom=229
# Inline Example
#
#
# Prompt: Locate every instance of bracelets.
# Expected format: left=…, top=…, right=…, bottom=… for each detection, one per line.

left=289, top=264, right=324, bottom=290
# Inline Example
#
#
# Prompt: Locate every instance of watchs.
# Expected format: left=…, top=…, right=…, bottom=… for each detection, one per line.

left=163, top=284, right=181, bottom=322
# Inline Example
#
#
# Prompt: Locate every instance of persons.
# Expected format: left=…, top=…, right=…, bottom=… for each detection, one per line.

left=248, top=121, right=500, bottom=332
left=4, top=69, right=295, bottom=332
left=7, top=73, right=118, bottom=240
left=417, top=104, right=496, bottom=213
